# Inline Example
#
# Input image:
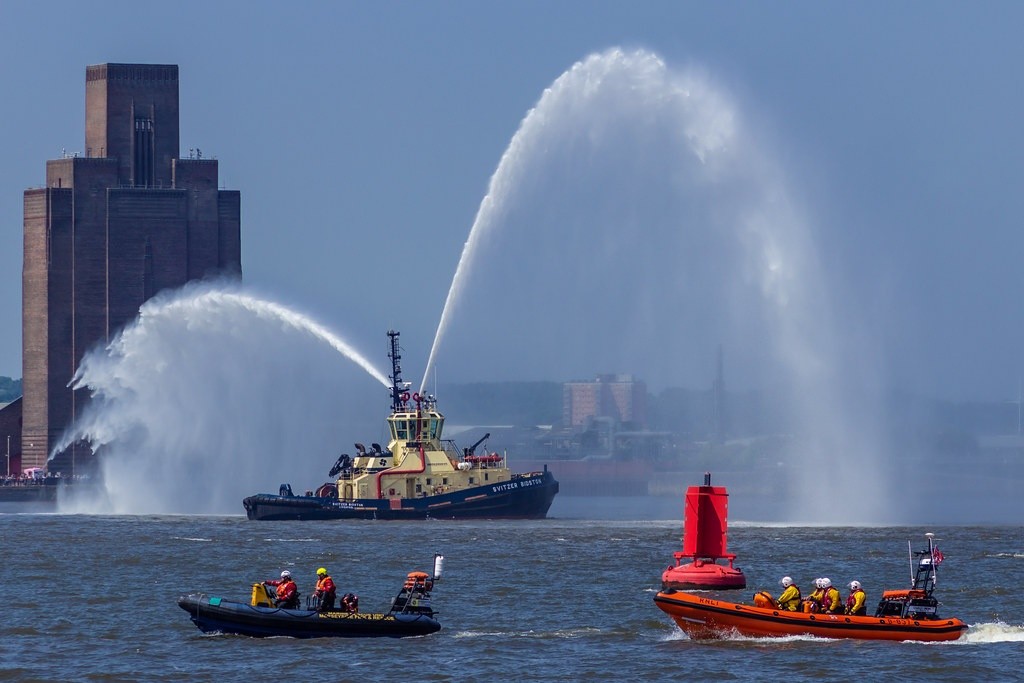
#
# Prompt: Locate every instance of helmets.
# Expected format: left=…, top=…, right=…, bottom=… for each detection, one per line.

left=281, top=569, right=291, bottom=577
left=823, top=578, right=832, bottom=588
left=317, top=568, right=328, bottom=576
left=851, top=581, right=862, bottom=590
left=816, top=578, right=823, bottom=588
left=782, top=576, right=793, bottom=587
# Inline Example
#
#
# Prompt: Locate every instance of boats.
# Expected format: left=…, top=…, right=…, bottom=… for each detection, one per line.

left=653, top=532, right=970, bottom=643
left=242, top=331, right=559, bottom=522
left=178, top=551, right=444, bottom=641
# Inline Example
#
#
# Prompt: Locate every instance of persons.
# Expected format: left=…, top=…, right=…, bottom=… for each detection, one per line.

left=260, top=569, right=297, bottom=610
left=802, top=577, right=846, bottom=615
left=844, top=581, right=867, bottom=615
left=776, top=576, right=802, bottom=613
left=312, top=567, right=336, bottom=612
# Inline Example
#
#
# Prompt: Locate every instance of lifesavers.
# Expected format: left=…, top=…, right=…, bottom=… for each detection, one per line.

left=404, top=392, right=410, bottom=400
left=403, top=580, right=431, bottom=589
left=243, top=497, right=254, bottom=511
left=413, top=392, right=419, bottom=401
